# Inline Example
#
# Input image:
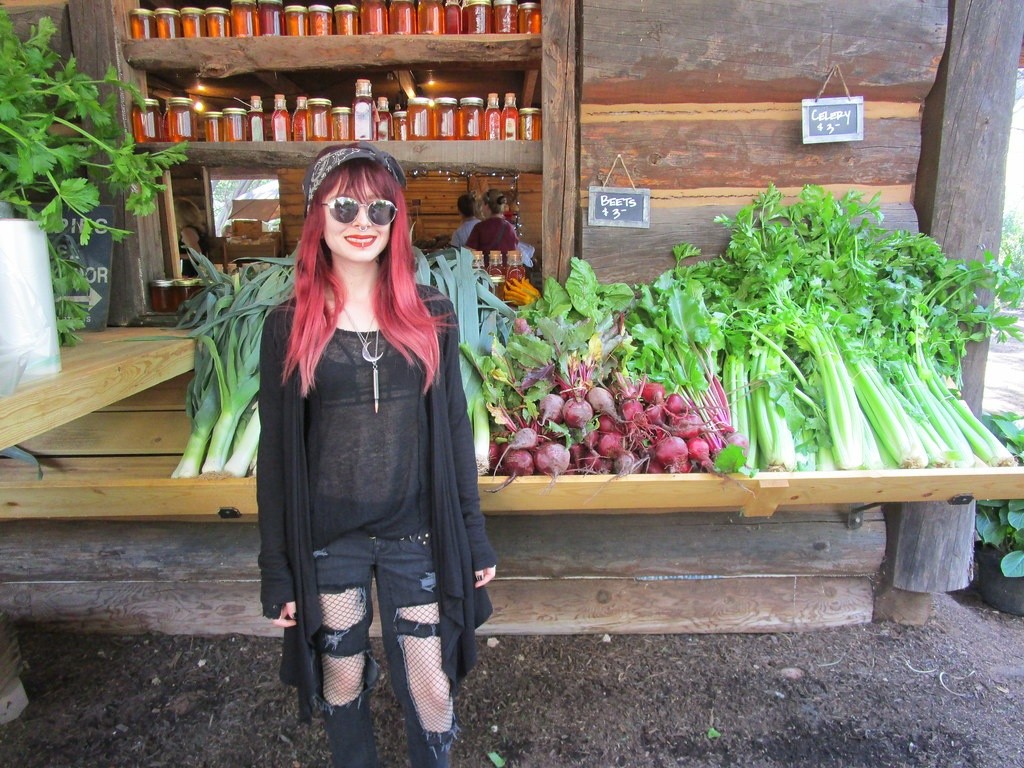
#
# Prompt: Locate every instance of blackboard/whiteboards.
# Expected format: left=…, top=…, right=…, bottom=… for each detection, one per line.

left=801, top=95, right=865, bottom=144
left=588, top=185, right=651, bottom=228
left=15, top=201, right=117, bottom=332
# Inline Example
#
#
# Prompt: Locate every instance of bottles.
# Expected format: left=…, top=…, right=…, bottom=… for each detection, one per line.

left=203, top=108, right=247, bottom=142
left=162, top=97, right=197, bottom=142
left=130, top=0, right=542, bottom=39
left=470, top=251, right=526, bottom=311
left=133, top=99, right=163, bottom=142
left=248, top=96, right=267, bottom=141
left=150, top=278, right=214, bottom=312
left=271, top=94, right=352, bottom=141
left=352, top=79, right=376, bottom=141
left=377, top=93, right=542, bottom=140
left=213, top=263, right=251, bottom=275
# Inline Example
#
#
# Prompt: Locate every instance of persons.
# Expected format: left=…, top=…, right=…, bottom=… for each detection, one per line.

left=450, top=194, right=483, bottom=250
left=255, top=143, right=498, bottom=768
left=173, top=198, right=210, bottom=278
left=464, top=189, right=520, bottom=269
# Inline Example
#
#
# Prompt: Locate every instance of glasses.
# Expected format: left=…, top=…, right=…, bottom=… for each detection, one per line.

left=319, top=196, right=397, bottom=226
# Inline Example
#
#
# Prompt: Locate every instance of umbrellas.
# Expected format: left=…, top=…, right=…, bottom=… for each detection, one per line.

left=228, top=180, right=281, bottom=224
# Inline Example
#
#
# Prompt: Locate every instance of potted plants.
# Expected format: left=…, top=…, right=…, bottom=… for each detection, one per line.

left=956, top=410, right=1024, bottom=618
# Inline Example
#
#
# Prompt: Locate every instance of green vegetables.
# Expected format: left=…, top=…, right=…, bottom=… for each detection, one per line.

left=0, top=7, right=187, bottom=346
left=668, top=179, right=1023, bottom=474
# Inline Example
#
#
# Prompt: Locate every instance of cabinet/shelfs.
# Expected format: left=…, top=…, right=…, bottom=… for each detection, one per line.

left=125, top=33, right=542, bottom=174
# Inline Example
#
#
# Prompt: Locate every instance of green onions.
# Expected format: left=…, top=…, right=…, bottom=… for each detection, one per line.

left=101, top=245, right=516, bottom=481
